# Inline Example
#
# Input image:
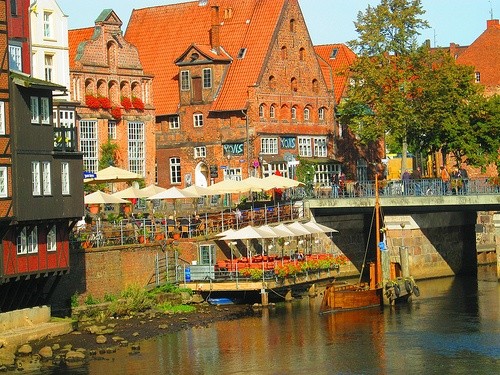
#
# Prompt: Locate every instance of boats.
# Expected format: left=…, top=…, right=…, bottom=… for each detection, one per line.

left=319, top=175, right=420, bottom=315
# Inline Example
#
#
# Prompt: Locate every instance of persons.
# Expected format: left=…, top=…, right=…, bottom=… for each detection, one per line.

left=330, top=165, right=469, bottom=195
left=89, top=207, right=305, bottom=260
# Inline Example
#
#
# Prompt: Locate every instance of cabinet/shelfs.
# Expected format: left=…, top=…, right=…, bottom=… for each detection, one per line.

left=200, top=244, right=216, bottom=265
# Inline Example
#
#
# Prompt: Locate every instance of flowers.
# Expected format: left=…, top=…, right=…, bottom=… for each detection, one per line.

left=85, top=94, right=145, bottom=121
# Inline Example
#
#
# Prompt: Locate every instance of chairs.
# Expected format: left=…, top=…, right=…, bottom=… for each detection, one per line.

left=147, top=205, right=291, bottom=237
left=218, top=253, right=332, bottom=269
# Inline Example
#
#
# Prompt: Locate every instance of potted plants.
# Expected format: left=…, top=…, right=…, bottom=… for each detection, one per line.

left=139, top=228, right=150, bottom=244
left=172, top=228, right=182, bottom=239
left=156, top=227, right=166, bottom=240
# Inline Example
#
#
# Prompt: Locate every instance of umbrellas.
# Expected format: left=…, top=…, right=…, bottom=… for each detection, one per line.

left=83, top=166, right=339, bottom=240
